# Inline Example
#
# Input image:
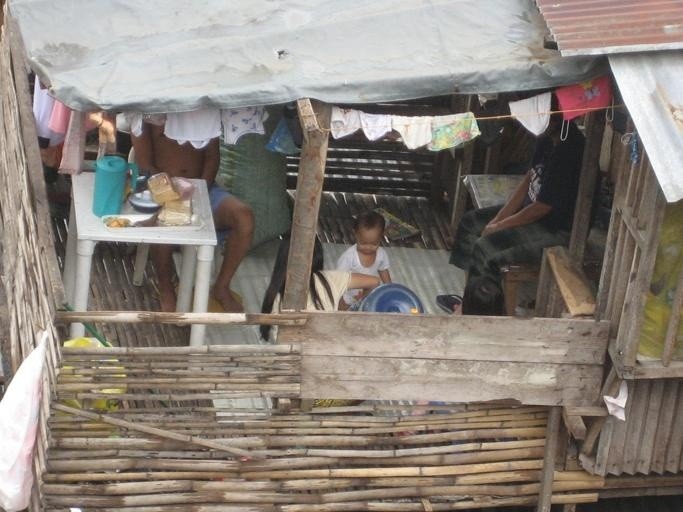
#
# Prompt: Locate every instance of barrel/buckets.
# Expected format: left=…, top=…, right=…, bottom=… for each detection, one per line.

left=345, top=284, right=423, bottom=314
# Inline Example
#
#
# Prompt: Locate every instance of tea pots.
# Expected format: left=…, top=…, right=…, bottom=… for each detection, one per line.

left=91, top=155, right=137, bottom=217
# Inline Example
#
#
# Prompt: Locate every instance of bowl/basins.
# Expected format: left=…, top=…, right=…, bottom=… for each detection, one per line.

left=126, top=190, right=163, bottom=213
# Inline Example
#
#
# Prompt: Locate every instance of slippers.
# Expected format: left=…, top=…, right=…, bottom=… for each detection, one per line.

left=436, top=293, right=464, bottom=314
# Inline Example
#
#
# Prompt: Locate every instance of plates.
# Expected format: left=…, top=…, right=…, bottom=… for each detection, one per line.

left=100, top=212, right=205, bottom=233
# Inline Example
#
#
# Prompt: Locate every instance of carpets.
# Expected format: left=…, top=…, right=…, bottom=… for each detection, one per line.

left=171, top=240, right=465, bottom=424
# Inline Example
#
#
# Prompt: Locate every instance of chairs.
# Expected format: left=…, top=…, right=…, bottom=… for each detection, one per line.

left=127, top=146, right=150, bottom=286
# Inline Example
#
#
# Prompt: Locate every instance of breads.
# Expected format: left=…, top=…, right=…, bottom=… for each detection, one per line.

left=171, top=177, right=193, bottom=198
left=147, top=173, right=179, bottom=203
left=157, top=200, right=192, bottom=224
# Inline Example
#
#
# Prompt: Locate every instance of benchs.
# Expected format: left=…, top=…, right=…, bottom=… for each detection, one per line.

left=460, top=174, right=606, bottom=315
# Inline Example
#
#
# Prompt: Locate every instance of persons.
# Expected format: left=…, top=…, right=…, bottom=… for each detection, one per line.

left=447, top=96, right=602, bottom=282
left=27, top=69, right=70, bottom=216
left=335, top=210, right=391, bottom=312
left=259, top=231, right=380, bottom=347
left=129, top=118, right=255, bottom=313
left=452, top=274, right=508, bottom=316
